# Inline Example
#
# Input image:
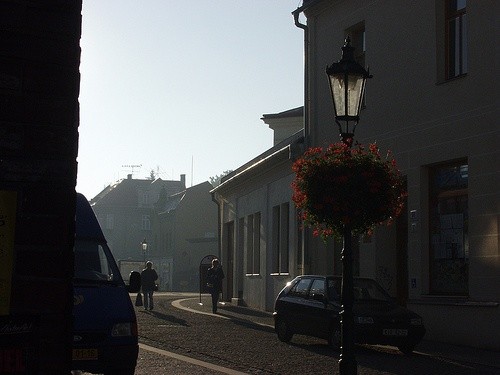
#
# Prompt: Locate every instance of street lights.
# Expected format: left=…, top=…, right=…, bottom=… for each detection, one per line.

left=324, top=34, right=371, bottom=375
left=142, top=238, right=148, bottom=269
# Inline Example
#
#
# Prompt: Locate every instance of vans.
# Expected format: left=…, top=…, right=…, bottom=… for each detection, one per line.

left=73, top=193, right=141, bottom=375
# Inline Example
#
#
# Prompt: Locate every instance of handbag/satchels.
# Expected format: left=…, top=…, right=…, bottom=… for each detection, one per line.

left=135, top=292, right=143, bottom=307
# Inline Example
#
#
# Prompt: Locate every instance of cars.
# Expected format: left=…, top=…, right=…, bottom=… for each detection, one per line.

left=272, top=275, right=424, bottom=353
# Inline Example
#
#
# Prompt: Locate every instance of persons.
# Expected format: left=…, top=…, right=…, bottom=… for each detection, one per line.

left=207, top=259, right=224, bottom=313
left=140, top=261, right=159, bottom=312
